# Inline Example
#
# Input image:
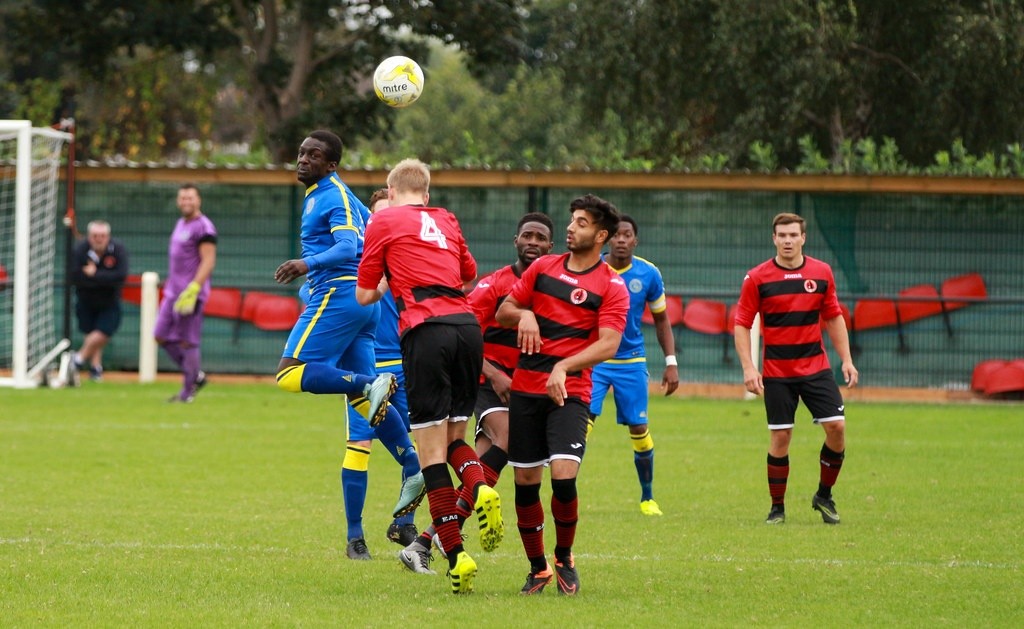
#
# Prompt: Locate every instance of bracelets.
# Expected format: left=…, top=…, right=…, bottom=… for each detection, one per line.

left=665, top=355, right=677, bottom=366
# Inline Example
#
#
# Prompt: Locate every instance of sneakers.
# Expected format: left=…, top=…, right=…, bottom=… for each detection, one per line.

left=811, top=491, right=840, bottom=524
left=432, top=528, right=468, bottom=560
left=386, top=523, right=419, bottom=546
left=191, top=371, right=207, bottom=395
left=392, top=468, right=425, bottom=519
left=362, top=372, right=398, bottom=427
left=87, top=362, right=104, bottom=383
left=519, top=561, right=554, bottom=596
left=554, top=551, right=580, bottom=596
left=65, top=350, right=83, bottom=388
left=399, top=539, right=437, bottom=576
left=345, top=536, right=373, bottom=561
left=166, top=393, right=193, bottom=404
left=473, top=485, right=504, bottom=552
left=446, top=551, right=478, bottom=597
left=640, top=499, right=663, bottom=517
left=766, top=510, right=785, bottom=526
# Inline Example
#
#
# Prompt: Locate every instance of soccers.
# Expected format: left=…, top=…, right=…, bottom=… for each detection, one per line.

left=373, top=56, right=424, bottom=107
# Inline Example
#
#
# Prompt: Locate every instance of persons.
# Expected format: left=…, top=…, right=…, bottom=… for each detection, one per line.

left=154, top=185, right=217, bottom=404
left=495, top=195, right=630, bottom=596
left=72, top=219, right=128, bottom=383
left=341, top=189, right=420, bottom=560
left=734, top=213, right=858, bottom=525
left=356, top=158, right=505, bottom=595
left=274, top=130, right=427, bottom=519
left=398, top=213, right=555, bottom=575
left=583, top=215, right=679, bottom=516
